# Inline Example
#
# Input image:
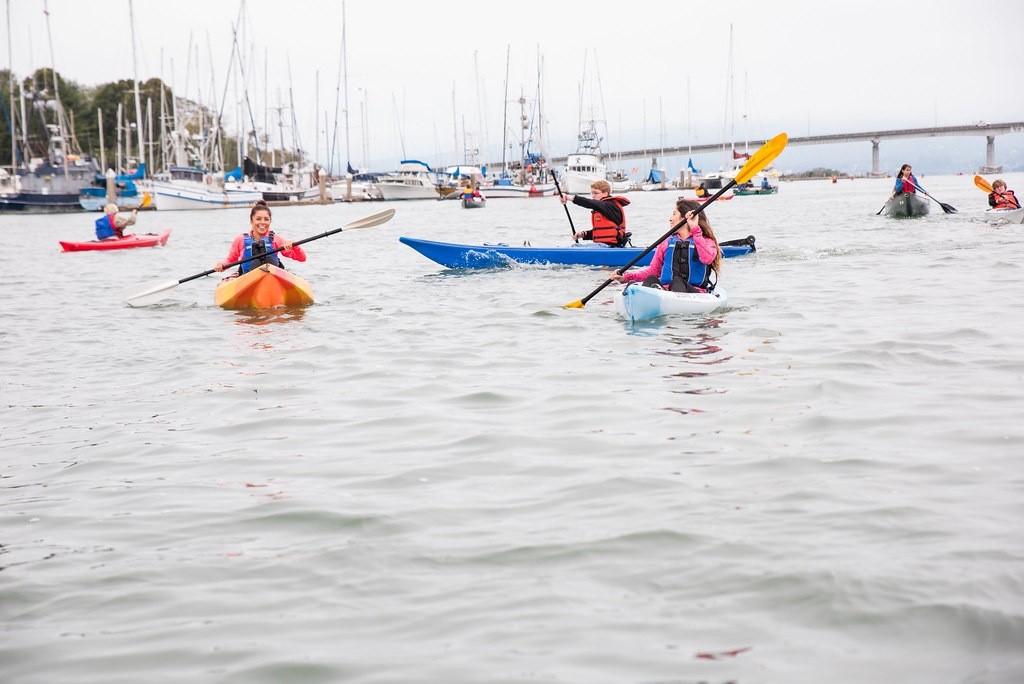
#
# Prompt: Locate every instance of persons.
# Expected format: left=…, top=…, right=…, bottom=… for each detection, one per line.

left=213, top=200, right=306, bottom=279
left=560, top=180, right=626, bottom=248
left=762, top=177, right=773, bottom=189
left=460, top=185, right=486, bottom=202
left=95, top=203, right=137, bottom=239
left=895, top=164, right=929, bottom=195
left=988, top=180, right=1021, bottom=209
left=738, top=179, right=754, bottom=188
left=610, top=200, right=721, bottom=293
left=697, top=182, right=712, bottom=198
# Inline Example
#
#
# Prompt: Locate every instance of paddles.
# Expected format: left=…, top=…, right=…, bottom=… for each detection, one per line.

left=900, top=175, right=957, bottom=213
left=876, top=190, right=896, bottom=215
left=119, top=208, right=396, bottom=302
left=551, top=169, right=579, bottom=244
left=974, top=176, right=1022, bottom=208
left=134, top=192, right=152, bottom=215
left=561, top=131, right=788, bottom=309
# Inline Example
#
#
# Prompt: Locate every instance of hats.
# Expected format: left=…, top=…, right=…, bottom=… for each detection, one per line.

left=104, top=204, right=118, bottom=214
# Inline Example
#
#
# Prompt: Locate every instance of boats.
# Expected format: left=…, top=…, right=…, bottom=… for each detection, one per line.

left=883, top=190, right=933, bottom=219
left=398, top=234, right=756, bottom=272
left=374, top=161, right=470, bottom=202
left=213, top=263, right=316, bottom=309
left=58, top=227, right=173, bottom=252
left=684, top=195, right=734, bottom=202
left=560, top=50, right=630, bottom=196
left=733, top=185, right=783, bottom=195
left=613, top=278, right=728, bottom=322
left=983, top=205, right=1024, bottom=226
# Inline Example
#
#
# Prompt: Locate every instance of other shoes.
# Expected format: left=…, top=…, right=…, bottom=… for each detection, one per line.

left=249, top=259, right=261, bottom=271
left=265, top=256, right=278, bottom=267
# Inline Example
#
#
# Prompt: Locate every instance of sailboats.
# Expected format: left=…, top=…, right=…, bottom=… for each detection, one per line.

left=1, top=0, right=376, bottom=214
left=640, top=98, right=669, bottom=190
left=477, top=42, right=555, bottom=199
left=699, top=22, right=781, bottom=191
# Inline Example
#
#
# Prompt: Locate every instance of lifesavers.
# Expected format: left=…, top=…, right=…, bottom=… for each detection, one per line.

left=206, top=176, right=212, bottom=185
